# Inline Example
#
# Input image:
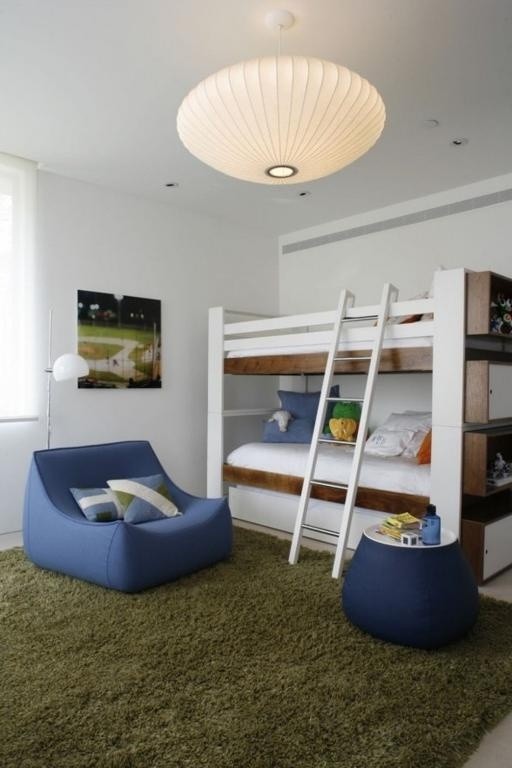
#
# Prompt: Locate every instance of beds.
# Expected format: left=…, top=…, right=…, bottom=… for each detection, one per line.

left=205, top=269, right=511, bottom=580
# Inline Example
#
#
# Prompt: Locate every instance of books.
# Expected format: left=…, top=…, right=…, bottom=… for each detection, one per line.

left=380, top=512, right=422, bottom=540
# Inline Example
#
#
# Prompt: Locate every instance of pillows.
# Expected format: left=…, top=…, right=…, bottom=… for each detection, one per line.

left=265, top=385, right=344, bottom=447
left=66, top=473, right=183, bottom=521
left=367, top=411, right=435, bottom=463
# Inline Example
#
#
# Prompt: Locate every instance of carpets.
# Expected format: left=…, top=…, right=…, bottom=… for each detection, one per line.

left=0, top=524, right=512, bottom=764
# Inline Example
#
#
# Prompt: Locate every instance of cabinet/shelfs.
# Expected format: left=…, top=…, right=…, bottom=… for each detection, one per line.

left=463, top=269, right=512, bottom=582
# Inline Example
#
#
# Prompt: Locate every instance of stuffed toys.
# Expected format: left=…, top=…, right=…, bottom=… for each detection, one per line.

left=324, top=402, right=370, bottom=447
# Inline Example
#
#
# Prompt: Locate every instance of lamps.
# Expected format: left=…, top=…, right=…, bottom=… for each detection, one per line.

left=45, top=305, right=93, bottom=451
left=176, top=10, right=392, bottom=185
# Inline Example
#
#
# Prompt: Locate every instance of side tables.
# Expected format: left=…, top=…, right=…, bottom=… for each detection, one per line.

left=343, top=523, right=477, bottom=647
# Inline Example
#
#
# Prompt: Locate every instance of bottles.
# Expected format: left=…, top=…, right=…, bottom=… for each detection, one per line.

left=420, top=504, right=440, bottom=545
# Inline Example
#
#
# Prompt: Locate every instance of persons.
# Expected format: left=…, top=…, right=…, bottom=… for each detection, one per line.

left=492, top=453, right=508, bottom=478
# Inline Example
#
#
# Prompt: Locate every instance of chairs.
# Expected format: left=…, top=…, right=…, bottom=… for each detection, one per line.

left=22, top=438, right=237, bottom=591
